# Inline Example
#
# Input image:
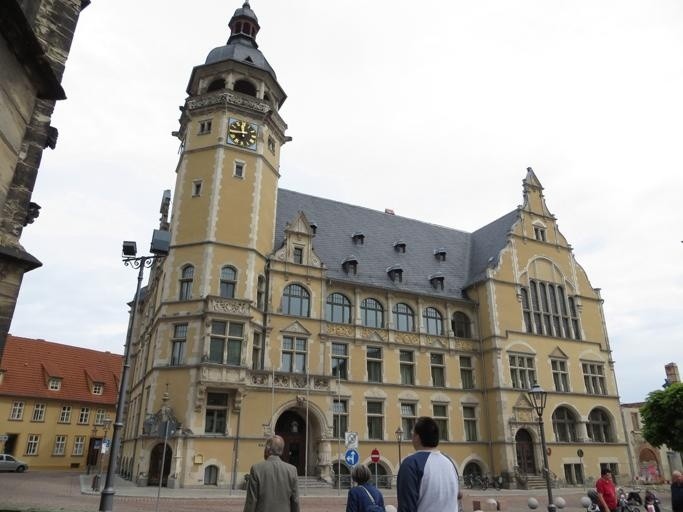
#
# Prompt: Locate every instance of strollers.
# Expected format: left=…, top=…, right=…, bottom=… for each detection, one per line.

left=617, top=486, right=643, bottom=512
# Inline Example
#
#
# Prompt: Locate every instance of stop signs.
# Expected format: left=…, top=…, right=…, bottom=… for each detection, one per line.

left=370, top=449, right=380, bottom=462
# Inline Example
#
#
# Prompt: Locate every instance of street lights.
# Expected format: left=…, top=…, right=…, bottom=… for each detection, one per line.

left=394, top=427, right=404, bottom=471
left=97, top=228, right=173, bottom=511
left=528, top=378, right=556, bottom=511
left=85, top=424, right=97, bottom=475
left=92, top=413, right=113, bottom=495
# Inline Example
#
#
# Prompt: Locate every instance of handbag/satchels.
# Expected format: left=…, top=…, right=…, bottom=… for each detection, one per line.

left=364, top=505, right=385, bottom=512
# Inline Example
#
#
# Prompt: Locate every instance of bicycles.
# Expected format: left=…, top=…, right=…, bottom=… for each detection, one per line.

left=463, top=470, right=504, bottom=490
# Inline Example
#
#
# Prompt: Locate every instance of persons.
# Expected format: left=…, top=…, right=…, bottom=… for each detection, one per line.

left=670, top=469, right=682, bottom=511
left=343, top=462, right=385, bottom=511
left=595, top=469, right=619, bottom=512
left=239, top=432, right=301, bottom=512
left=394, top=412, right=462, bottom=511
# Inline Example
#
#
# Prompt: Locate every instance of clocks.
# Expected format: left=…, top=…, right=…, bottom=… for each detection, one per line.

left=225, top=116, right=260, bottom=153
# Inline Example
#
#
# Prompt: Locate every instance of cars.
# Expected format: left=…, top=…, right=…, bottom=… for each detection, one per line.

left=0, top=453, right=28, bottom=473
